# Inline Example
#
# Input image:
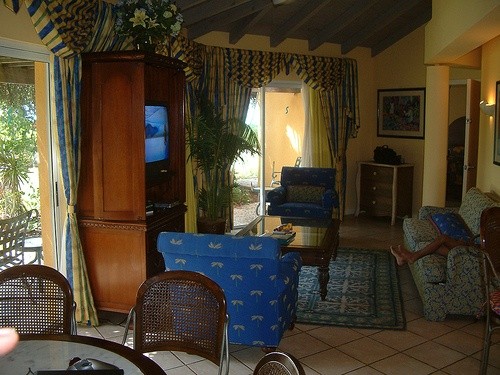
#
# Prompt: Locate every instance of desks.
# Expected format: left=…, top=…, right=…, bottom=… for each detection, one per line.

left=0, top=333, right=167, bottom=375
left=235, top=215, right=342, bottom=301
left=254, top=187, right=275, bottom=216
left=18, top=238, right=43, bottom=265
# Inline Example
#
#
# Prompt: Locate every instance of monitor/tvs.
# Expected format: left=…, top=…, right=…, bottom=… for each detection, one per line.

left=144, top=105, right=172, bottom=179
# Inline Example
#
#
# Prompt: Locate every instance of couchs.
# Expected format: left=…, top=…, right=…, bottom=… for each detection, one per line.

left=402, top=187, right=500, bottom=322
left=155, top=231, right=300, bottom=352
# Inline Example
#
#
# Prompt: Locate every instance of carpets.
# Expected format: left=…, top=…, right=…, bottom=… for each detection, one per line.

left=293, top=246, right=408, bottom=331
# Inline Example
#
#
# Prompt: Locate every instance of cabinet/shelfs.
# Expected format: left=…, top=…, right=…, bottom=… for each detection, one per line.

left=354, top=162, right=416, bottom=226
left=74, top=204, right=187, bottom=326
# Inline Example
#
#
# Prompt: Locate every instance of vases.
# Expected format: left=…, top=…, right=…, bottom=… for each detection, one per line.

left=134, top=35, right=151, bottom=50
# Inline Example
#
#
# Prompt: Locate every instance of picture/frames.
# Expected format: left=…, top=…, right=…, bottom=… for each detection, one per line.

left=376, top=88, right=425, bottom=140
left=492, top=80, right=500, bottom=167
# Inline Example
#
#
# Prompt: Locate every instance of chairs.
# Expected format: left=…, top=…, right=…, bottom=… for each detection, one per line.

left=0, top=264, right=78, bottom=336
left=267, top=167, right=339, bottom=220
left=0, top=208, right=39, bottom=269
left=253, top=351, right=305, bottom=375
left=270, top=157, right=301, bottom=188
left=122, top=269, right=230, bottom=375
left=478, top=207, right=500, bottom=375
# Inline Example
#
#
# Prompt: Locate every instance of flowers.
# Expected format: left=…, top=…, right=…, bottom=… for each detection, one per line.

left=111, top=0, right=183, bottom=38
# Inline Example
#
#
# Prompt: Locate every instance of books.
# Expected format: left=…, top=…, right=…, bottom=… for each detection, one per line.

left=271, top=232, right=296, bottom=245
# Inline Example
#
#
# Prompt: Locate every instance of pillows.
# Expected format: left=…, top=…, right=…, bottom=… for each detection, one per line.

left=433, top=212, right=476, bottom=243
left=286, top=185, right=327, bottom=207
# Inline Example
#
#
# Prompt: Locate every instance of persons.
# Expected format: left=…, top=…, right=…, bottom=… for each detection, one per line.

left=390, top=234, right=481, bottom=265
